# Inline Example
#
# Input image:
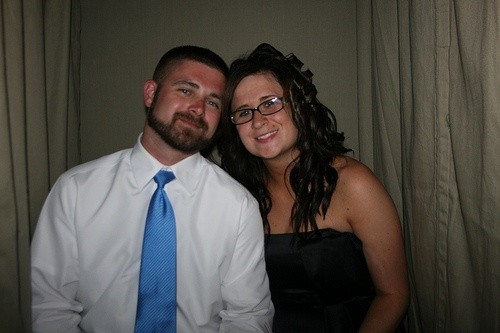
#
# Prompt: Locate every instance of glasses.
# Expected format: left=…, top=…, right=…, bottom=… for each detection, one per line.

left=230, top=96, right=289, bottom=125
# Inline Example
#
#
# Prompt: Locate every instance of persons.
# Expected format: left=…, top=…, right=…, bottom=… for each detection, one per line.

left=30, top=46, right=275, bottom=333
left=219, top=44, right=410, bottom=333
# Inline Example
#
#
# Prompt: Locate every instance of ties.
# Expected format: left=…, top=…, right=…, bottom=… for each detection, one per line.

left=135, top=169, right=177, bottom=333
